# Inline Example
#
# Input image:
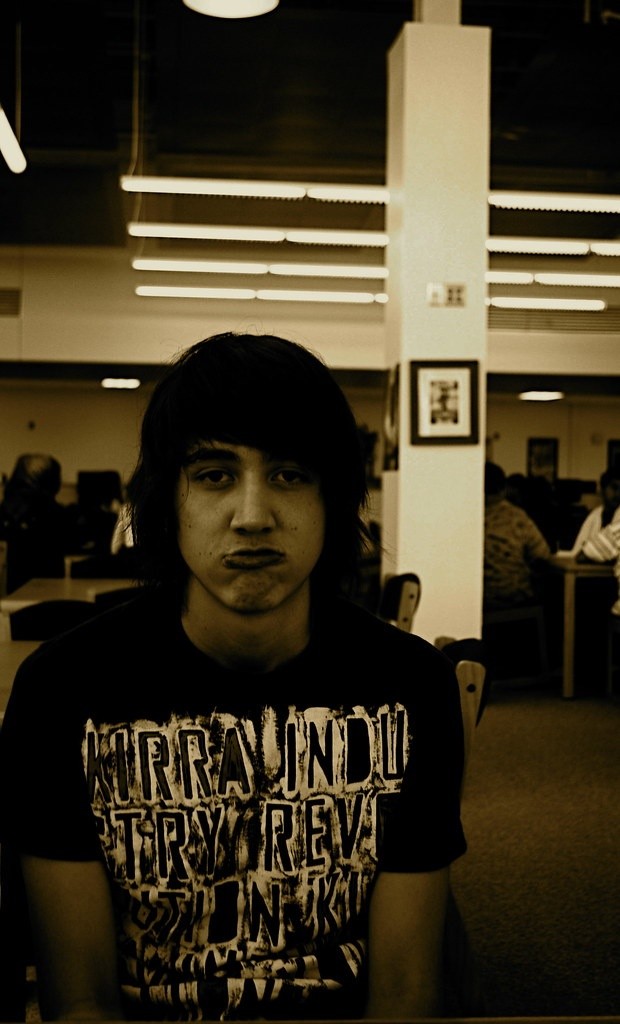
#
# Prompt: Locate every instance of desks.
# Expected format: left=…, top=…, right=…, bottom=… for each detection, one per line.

left=0, top=573, right=155, bottom=729
left=544, top=549, right=612, bottom=702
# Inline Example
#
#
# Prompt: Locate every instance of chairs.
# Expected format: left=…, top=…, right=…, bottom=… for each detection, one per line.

left=373, top=569, right=422, bottom=632
left=429, top=631, right=498, bottom=802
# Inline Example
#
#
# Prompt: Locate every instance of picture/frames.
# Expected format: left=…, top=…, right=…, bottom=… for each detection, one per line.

left=407, top=358, right=484, bottom=460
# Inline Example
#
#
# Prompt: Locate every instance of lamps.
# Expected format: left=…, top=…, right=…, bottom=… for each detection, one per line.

left=120, top=0, right=620, bottom=307
left=0, top=0, right=29, bottom=178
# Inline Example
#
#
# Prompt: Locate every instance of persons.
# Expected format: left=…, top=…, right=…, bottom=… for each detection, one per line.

left=483, top=456, right=620, bottom=685
left=0, top=334, right=469, bottom=1024
